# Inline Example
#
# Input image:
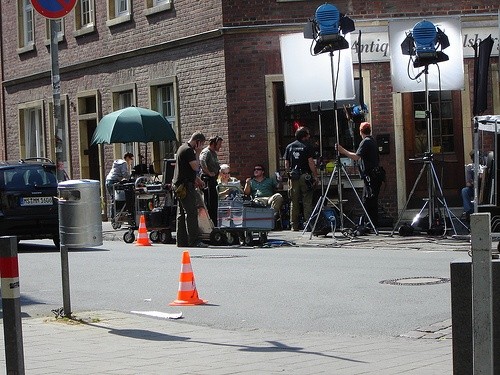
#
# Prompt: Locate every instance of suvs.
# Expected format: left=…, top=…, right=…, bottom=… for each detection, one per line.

left=0, top=157, right=70, bottom=251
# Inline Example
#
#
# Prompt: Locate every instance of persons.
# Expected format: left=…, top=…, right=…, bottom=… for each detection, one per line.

left=104, top=152, right=135, bottom=223
left=285, top=126, right=320, bottom=232
left=199, top=136, right=223, bottom=245
left=462, top=150, right=485, bottom=219
left=244, top=164, right=283, bottom=221
left=172, top=131, right=207, bottom=247
left=335, top=122, right=384, bottom=230
left=215, top=164, right=244, bottom=243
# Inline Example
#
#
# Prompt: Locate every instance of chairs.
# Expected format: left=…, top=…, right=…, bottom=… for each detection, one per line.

left=28, top=171, right=44, bottom=185
left=10, top=173, right=26, bottom=186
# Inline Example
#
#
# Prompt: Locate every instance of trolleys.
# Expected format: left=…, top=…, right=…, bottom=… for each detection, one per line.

left=212, top=184, right=275, bottom=245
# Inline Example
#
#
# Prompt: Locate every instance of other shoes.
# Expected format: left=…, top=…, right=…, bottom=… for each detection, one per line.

left=462, top=212, right=466, bottom=216
left=189, top=242, right=209, bottom=247
left=291, top=226, right=299, bottom=231
left=176, top=241, right=187, bottom=247
left=306, top=226, right=312, bottom=231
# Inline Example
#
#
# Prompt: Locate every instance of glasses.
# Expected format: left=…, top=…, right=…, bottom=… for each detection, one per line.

left=220, top=171, right=231, bottom=175
left=253, top=168, right=263, bottom=171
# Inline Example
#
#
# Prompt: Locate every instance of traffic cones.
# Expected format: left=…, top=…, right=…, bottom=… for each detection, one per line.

left=168, top=251, right=208, bottom=306
left=133, top=215, right=153, bottom=246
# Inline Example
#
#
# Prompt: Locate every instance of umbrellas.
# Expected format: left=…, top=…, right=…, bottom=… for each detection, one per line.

left=89, top=107, right=178, bottom=161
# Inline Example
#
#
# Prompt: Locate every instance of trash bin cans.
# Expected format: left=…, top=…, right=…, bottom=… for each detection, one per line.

left=57, top=178, right=104, bottom=249
